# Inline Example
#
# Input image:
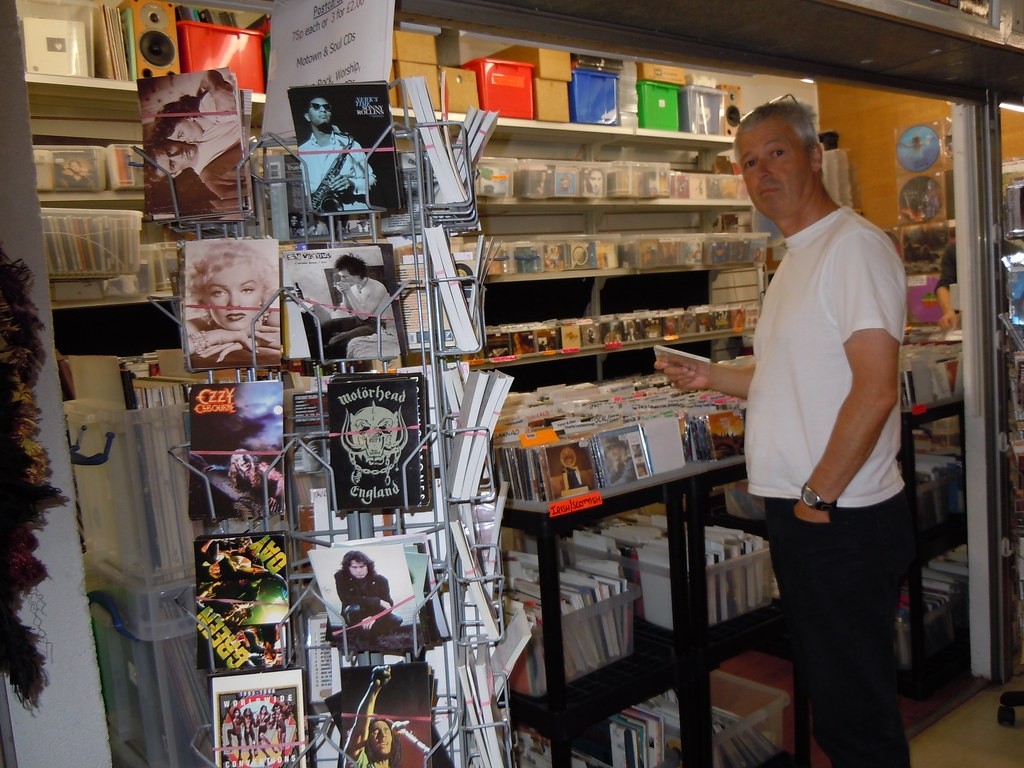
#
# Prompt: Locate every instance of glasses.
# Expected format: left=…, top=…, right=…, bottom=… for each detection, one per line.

left=738, top=93, right=816, bottom=135
left=307, top=104, right=332, bottom=112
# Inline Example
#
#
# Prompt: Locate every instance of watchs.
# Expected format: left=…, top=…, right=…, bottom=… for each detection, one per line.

left=802, top=482, right=837, bottom=511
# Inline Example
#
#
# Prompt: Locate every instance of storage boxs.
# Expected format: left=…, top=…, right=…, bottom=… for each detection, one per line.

left=16, top=0, right=968, bottom=768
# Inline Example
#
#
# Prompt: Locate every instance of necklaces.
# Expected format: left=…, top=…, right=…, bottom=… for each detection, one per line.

left=357, top=278, right=369, bottom=293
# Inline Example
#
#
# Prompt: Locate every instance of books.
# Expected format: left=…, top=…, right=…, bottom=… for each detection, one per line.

left=93, top=6, right=788, bottom=768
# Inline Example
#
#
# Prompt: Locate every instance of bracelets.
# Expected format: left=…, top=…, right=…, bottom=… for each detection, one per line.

left=191, top=331, right=208, bottom=354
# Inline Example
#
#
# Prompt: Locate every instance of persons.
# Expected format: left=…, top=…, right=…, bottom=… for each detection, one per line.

left=150, top=89, right=241, bottom=140
left=184, top=240, right=280, bottom=365
left=146, top=70, right=250, bottom=200
left=343, top=665, right=404, bottom=768
left=298, top=97, right=376, bottom=212
left=655, top=101, right=916, bottom=768
left=935, top=230, right=1024, bottom=331
left=333, top=551, right=404, bottom=632
left=317, top=255, right=389, bottom=361
left=204, top=448, right=283, bottom=513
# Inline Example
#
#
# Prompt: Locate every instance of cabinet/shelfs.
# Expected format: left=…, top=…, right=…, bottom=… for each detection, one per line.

left=478, top=400, right=964, bottom=767
left=20, top=72, right=765, bottom=379
